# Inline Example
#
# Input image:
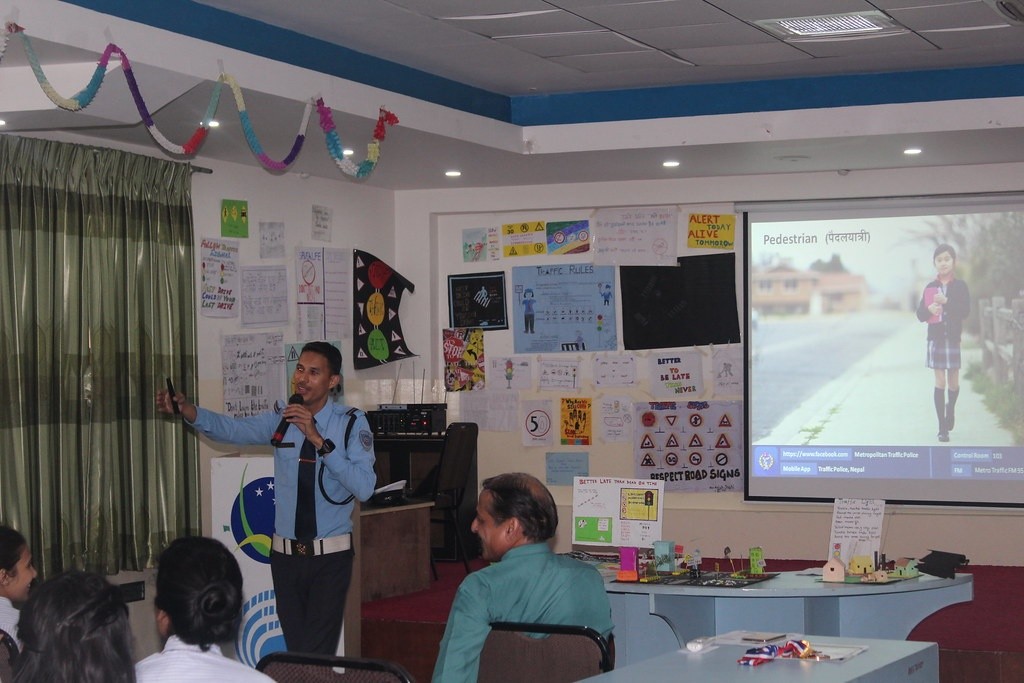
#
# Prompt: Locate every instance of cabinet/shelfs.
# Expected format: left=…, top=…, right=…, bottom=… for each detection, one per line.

left=364, top=422, right=483, bottom=560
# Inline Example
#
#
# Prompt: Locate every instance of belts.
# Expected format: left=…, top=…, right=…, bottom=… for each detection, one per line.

left=272, top=533, right=351, bottom=558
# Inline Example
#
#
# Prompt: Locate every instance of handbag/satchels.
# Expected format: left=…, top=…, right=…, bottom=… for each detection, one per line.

left=914, top=549, right=969, bottom=580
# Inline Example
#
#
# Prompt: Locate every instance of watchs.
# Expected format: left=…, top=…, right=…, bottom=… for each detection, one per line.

left=317, top=439, right=335, bottom=456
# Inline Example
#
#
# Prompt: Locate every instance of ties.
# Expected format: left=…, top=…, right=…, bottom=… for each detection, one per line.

left=294, top=417, right=317, bottom=546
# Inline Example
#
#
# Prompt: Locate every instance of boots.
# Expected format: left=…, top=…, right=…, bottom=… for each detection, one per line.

left=946, top=389, right=959, bottom=431
left=934, top=387, right=949, bottom=442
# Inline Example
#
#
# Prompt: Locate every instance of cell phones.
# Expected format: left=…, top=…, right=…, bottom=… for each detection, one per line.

left=741, top=632, right=785, bottom=643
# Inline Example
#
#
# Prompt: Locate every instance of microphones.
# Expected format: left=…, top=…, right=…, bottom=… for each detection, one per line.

left=270, top=393, right=304, bottom=447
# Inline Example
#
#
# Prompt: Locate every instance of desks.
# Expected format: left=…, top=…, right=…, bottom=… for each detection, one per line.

left=574, top=629, right=941, bottom=681
left=580, top=558, right=975, bottom=670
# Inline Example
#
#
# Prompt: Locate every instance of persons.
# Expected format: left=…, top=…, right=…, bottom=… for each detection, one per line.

left=0, top=522, right=39, bottom=681
left=134, top=535, right=277, bottom=682
left=14, top=570, right=137, bottom=682
left=158, top=341, right=377, bottom=656
left=917, top=244, right=971, bottom=442
left=431, top=472, right=615, bottom=682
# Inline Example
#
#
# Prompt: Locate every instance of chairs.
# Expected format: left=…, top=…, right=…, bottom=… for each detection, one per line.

left=1, top=627, right=23, bottom=682
left=404, top=422, right=479, bottom=579
left=255, top=652, right=414, bottom=682
left=477, top=622, right=614, bottom=683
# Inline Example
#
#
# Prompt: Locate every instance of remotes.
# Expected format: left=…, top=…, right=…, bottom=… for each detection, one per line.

left=166, top=377, right=180, bottom=414
left=686, top=636, right=714, bottom=652
